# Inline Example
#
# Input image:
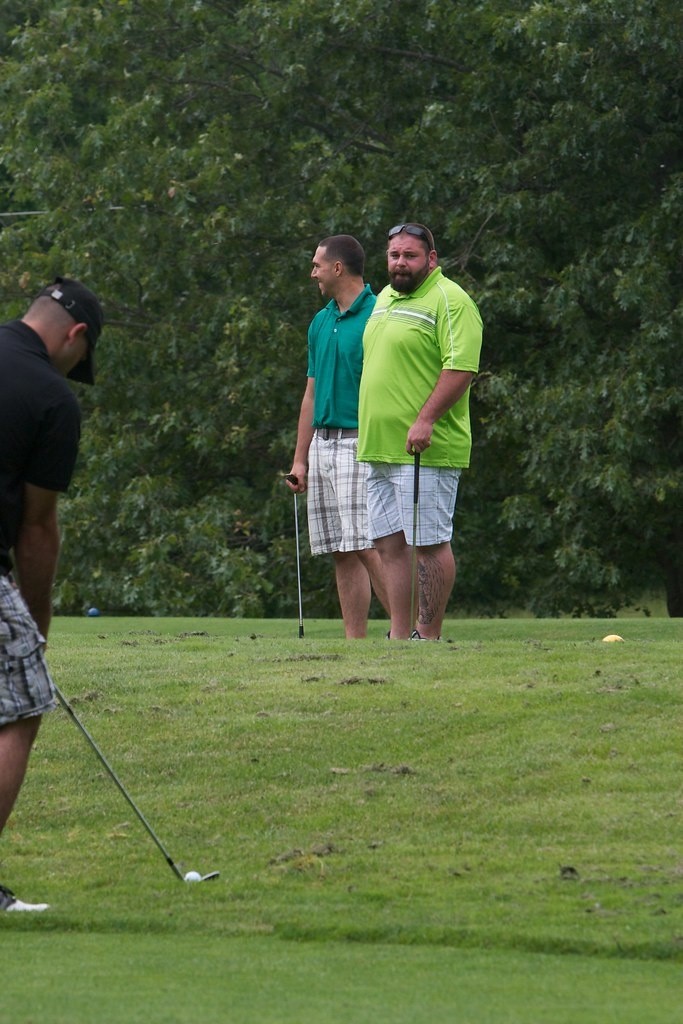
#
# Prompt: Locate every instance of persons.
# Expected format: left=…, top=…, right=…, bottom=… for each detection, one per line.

left=356, top=222, right=482, bottom=640
left=285, top=235, right=391, bottom=640
left=0, top=280, right=103, bottom=912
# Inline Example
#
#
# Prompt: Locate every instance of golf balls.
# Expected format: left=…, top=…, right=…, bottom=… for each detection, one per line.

left=185, top=871, right=201, bottom=883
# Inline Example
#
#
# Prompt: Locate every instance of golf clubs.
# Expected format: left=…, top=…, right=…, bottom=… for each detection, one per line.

left=293, top=491, right=305, bottom=639
left=52, top=684, right=221, bottom=883
left=409, top=449, right=421, bottom=641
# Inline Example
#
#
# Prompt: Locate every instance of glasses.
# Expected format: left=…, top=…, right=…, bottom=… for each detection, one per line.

left=388, top=224, right=432, bottom=251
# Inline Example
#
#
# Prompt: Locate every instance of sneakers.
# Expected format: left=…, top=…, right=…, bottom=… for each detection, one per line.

left=0, top=883, right=51, bottom=912
left=384, top=630, right=391, bottom=640
left=408, top=629, right=442, bottom=641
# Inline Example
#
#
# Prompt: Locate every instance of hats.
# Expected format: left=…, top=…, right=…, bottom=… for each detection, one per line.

left=31, top=277, right=103, bottom=386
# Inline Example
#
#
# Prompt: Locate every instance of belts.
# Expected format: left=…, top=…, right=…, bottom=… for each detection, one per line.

left=316, top=428, right=358, bottom=440
